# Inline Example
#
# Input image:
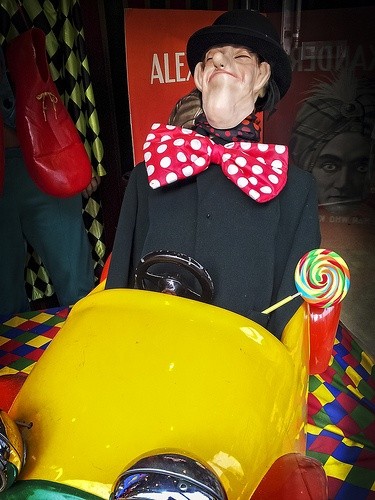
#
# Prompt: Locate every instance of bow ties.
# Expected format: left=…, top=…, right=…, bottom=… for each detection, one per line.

left=143, top=123, right=290, bottom=204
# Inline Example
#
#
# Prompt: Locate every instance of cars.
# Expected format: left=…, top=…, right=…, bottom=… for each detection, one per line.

left=0, top=249, right=341, bottom=500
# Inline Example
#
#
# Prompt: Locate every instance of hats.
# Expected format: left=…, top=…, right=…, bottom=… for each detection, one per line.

left=186, top=9, right=293, bottom=109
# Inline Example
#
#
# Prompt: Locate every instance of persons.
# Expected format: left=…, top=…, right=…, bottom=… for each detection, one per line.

left=99, top=7, right=321, bottom=348
left=0, top=28, right=98, bottom=320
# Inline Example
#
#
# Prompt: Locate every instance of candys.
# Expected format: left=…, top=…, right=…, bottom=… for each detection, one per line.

left=261, top=248, right=351, bottom=315
